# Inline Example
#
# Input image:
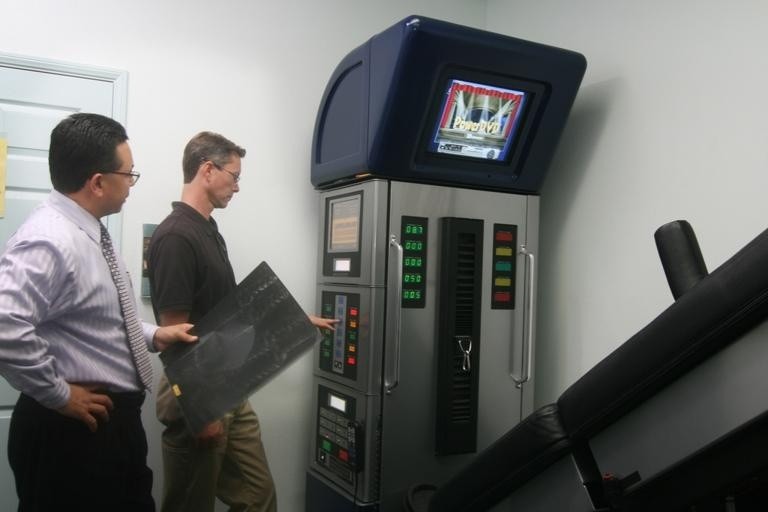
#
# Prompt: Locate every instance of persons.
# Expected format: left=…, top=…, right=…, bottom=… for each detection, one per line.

left=146, top=130, right=343, bottom=512
left=0, top=110, right=199, bottom=511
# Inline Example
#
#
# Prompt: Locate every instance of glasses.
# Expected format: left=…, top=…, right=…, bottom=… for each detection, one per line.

left=203, top=158, right=242, bottom=183
left=97, top=166, right=142, bottom=187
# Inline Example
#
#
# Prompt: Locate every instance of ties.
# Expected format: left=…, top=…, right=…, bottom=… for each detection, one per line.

left=97, top=222, right=155, bottom=395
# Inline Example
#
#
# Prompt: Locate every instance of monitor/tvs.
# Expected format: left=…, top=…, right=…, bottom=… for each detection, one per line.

left=412, top=62, right=545, bottom=185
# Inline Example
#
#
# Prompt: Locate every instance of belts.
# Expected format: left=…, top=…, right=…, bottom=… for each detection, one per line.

left=100, top=390, right=147, bottom=409
